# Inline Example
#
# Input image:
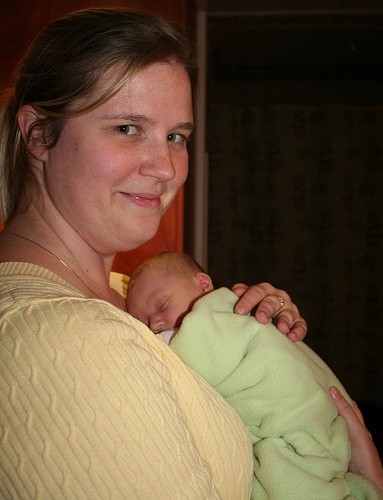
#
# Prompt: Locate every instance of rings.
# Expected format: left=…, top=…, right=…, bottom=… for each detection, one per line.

left=268, top=294, right=284, bottom=307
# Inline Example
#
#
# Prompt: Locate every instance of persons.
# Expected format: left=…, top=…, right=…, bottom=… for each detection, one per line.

left=0, top=6, right=383, bottom=500
left=126, top=251, right=352, bottom=482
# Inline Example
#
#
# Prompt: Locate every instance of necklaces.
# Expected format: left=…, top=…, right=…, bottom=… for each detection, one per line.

left=1, top=231, right=126, bottom=313
